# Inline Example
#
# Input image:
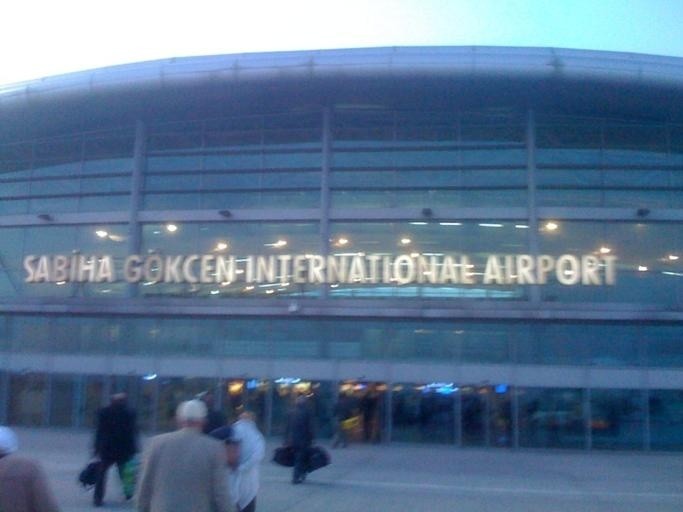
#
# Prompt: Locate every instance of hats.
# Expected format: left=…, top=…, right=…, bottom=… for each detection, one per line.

left=0, top=426, right=19, bottom=455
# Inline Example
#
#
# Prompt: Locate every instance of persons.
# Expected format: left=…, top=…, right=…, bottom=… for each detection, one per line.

left=93, top=392, right=141, bottom=507
left=135, top=394, right=265, bottom=512
left=284, top=394, right=313, bottom=484
left=332, top=391, right=356, bottom=449
left=0, top=427, right=61, bottom=512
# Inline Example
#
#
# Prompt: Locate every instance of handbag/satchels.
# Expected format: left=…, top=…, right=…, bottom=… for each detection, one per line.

left=306, top=446, right=331, bottom=472
left=80, top=462, right=105, bottom=485
left=275, top=447, right=295, bottom=466
